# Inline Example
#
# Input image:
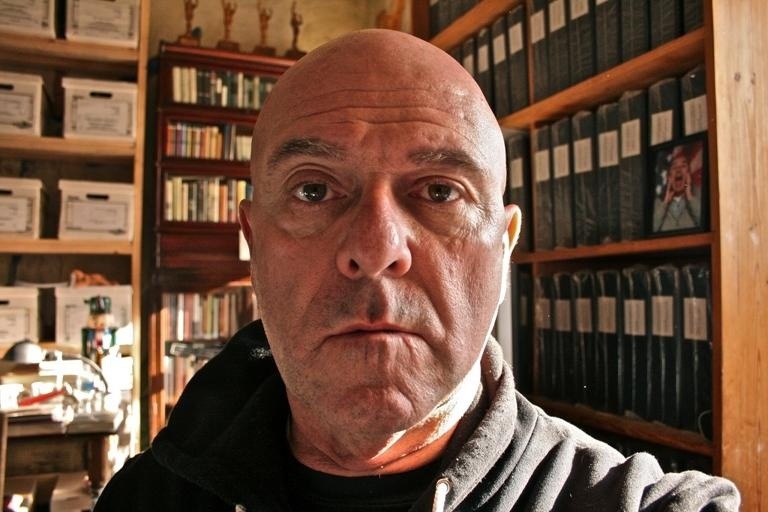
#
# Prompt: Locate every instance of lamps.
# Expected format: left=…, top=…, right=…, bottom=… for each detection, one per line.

left=0, top=332, right=118, bottom=400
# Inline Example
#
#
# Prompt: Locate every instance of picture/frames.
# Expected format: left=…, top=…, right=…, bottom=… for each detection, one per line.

left=640, top=126, right=708, bottom=240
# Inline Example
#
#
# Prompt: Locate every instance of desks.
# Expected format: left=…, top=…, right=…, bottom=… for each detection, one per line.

left=0, top=366, right=124, bottom=496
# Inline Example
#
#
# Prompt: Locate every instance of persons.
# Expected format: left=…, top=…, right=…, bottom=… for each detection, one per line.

left=89, top=28, right=742, bottom=511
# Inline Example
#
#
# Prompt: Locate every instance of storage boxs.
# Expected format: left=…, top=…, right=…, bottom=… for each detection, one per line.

left=0, top=69, right=57, bottom=139
left=0, top=174, right=48, bottom=239
left=55, top=176, right=135, bottom=242
left=1, top=2, right=58, bottom=41
left=2, top=284, right=42, bottom=346
left=64, top=1, right=141, bottom=50
left=52, top=284, right=136, bottom=347
left=61, top=74, right=140, bottom=143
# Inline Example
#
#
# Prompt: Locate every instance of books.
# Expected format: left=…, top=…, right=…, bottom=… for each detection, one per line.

left=528, top=260, right=716, bottom=433
left=163, top=59, right=275, bottom=229
left=505, top=73, right=710, bottom=247
left=445, top=2, right=704, bottom=116
left=161, top=288, right=259, bottom=420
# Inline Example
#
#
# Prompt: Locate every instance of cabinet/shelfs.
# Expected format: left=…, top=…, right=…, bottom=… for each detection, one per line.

left=143, top=33, right=304, bottom=446
left=0, top=2, right=149, bottom=472
left=407, top=0, right=768, bottom=511
